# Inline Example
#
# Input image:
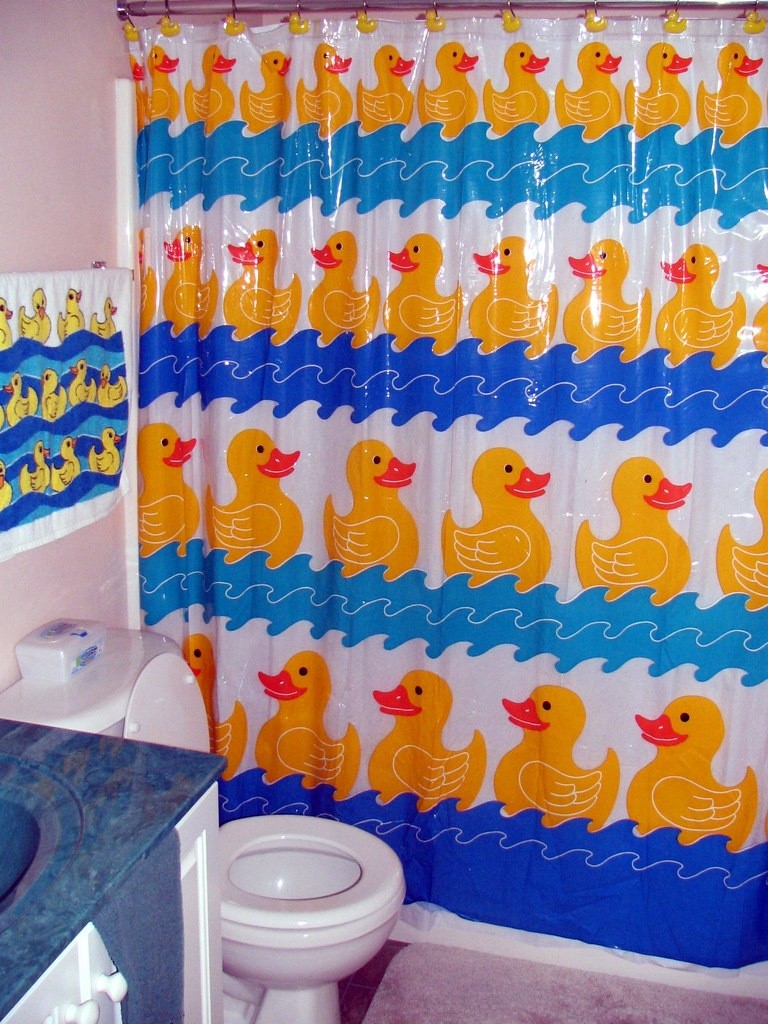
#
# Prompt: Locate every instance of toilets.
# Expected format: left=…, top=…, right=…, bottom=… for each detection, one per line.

left=0, top=622, right=407, bottom=1024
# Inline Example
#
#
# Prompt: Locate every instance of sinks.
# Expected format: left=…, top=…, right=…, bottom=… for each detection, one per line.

left=0, top=752, right=85, bottom=933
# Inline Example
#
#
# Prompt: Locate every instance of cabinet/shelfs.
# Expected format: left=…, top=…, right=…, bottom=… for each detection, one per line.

left=0, top=781, right=224, bottom=1024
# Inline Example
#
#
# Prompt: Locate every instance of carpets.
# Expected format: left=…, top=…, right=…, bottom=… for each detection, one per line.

left=363, top=942, right=768, bottom=1024
